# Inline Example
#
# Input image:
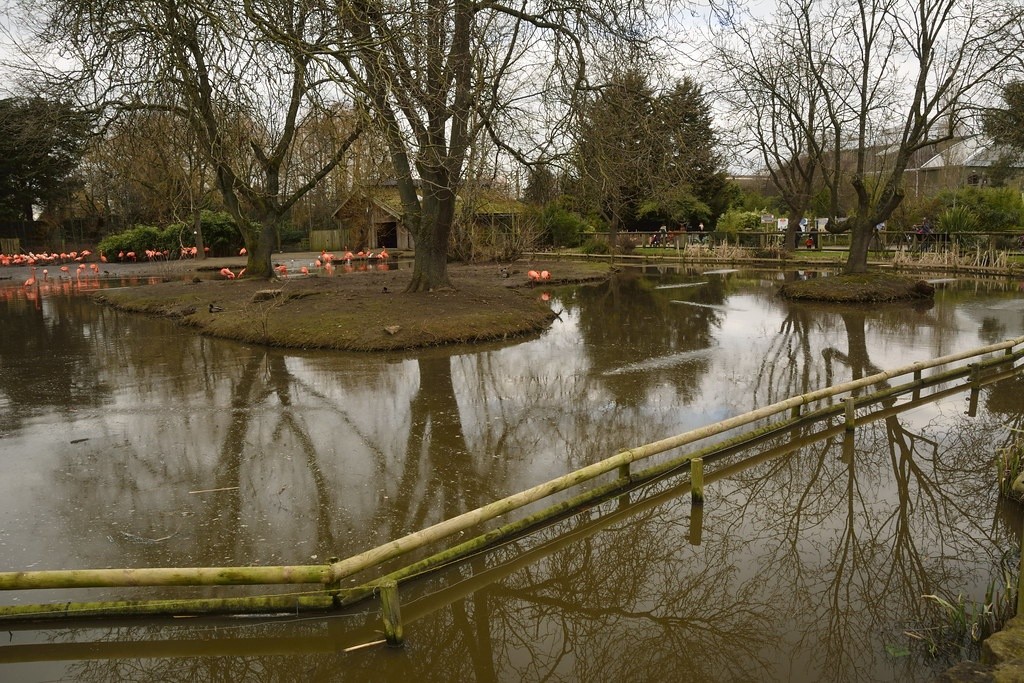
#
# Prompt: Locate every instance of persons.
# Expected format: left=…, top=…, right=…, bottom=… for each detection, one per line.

left=698, top=222, right=705, bottom=244
left=806, top=228, right=818, bottom=250
left=648, top=230, right=675, bottom=246
left=795, top=225, right=802, bottom=249
left=919, top=221, right=931, bottom=247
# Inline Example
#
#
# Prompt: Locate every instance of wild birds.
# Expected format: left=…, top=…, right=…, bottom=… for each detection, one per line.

left=527, top=269, right=553, bottom=289
left=0, top=242, right=390, bottom=286
